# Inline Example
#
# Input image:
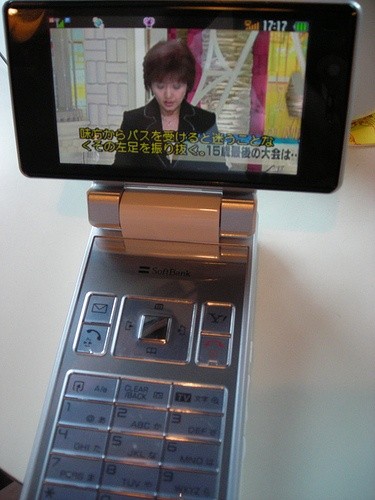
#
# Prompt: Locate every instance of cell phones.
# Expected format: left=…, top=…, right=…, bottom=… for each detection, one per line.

left=1, top=0, right=367, bottom=195
left=19, top=180, right=260, bottom=499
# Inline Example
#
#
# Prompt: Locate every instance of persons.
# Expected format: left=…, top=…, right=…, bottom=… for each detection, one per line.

left=112, top=41, right=229, bottom=171
